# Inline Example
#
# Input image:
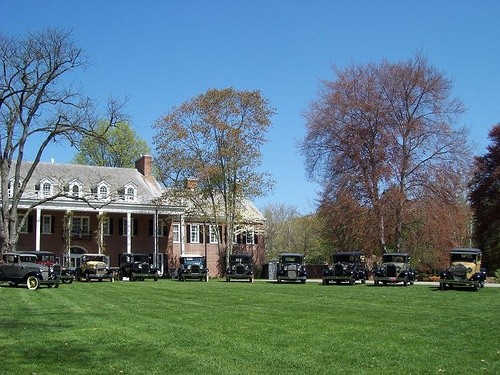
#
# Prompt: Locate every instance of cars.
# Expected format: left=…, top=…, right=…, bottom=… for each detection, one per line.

left=119, top=252, right=162, bottom=283
left=74, top=254, right=115, bottom=282
left=276, top=253, right=307, bottom=284
left=177, top=253, right=209, bottom=282
left=16, top=251, right=75, bottom=285
left=374, top=253, right=415, bottom=286
left=226, top=253, right=255, bottom=283
left=321, top=252, right=366, bottom=286
left=0, top=252, right=61, bottom=292
left=439, top=248, right=487, bottom=290
